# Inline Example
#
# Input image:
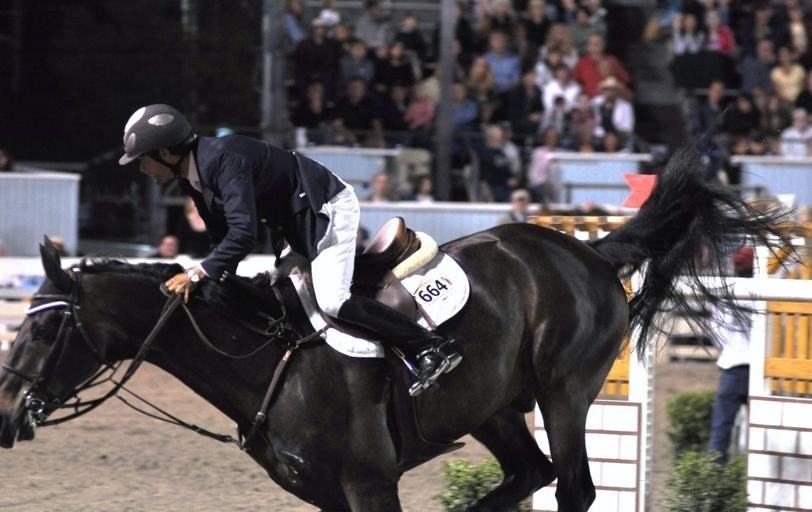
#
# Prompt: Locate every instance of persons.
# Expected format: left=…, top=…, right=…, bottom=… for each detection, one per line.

left=147, top=231, right=182, bottom=258
left=283, top=0, right=812, bottom=201
left=117, top=103, right=466, bottom=400
left=703, top=244, right=756, bottom=462
left=174, top=195, right=210, bottom=258
left=498, top=188, right=532, bottom=226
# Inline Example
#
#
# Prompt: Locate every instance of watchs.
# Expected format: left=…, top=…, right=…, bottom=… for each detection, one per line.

left=184, top=266, right=202, bottom=285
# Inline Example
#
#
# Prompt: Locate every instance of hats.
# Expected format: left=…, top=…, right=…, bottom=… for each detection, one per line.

left=735, top=248, right=753, bottom=270
left=597, top=76, right=625, bottom=91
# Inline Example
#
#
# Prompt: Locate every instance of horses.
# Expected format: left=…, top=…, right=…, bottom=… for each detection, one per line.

left=0, top=106, right=808, bottom=512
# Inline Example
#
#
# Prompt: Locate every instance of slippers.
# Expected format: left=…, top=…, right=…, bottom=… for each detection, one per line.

left=338, top=295, right=463, bottom=396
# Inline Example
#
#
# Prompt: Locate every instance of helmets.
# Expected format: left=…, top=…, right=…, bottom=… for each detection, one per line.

left=119, top=104, right=192, bottom=166
